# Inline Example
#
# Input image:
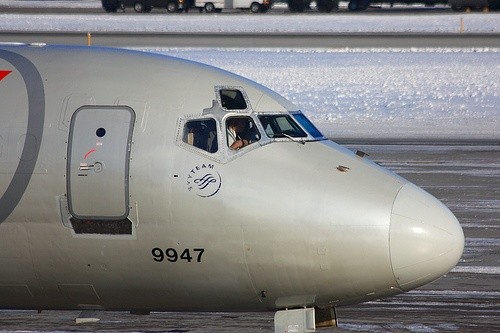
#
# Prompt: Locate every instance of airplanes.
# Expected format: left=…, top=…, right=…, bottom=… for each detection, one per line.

left=0, top=44, right=467, bottom=315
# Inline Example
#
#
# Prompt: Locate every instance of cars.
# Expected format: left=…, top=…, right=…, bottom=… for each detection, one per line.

left=103, top=1, right=500, bottom=13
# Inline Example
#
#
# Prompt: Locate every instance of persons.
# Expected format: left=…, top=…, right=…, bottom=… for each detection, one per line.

left=247, top=118, right=270, bottom=141
left=227, top=118, right=248, bottom=149
